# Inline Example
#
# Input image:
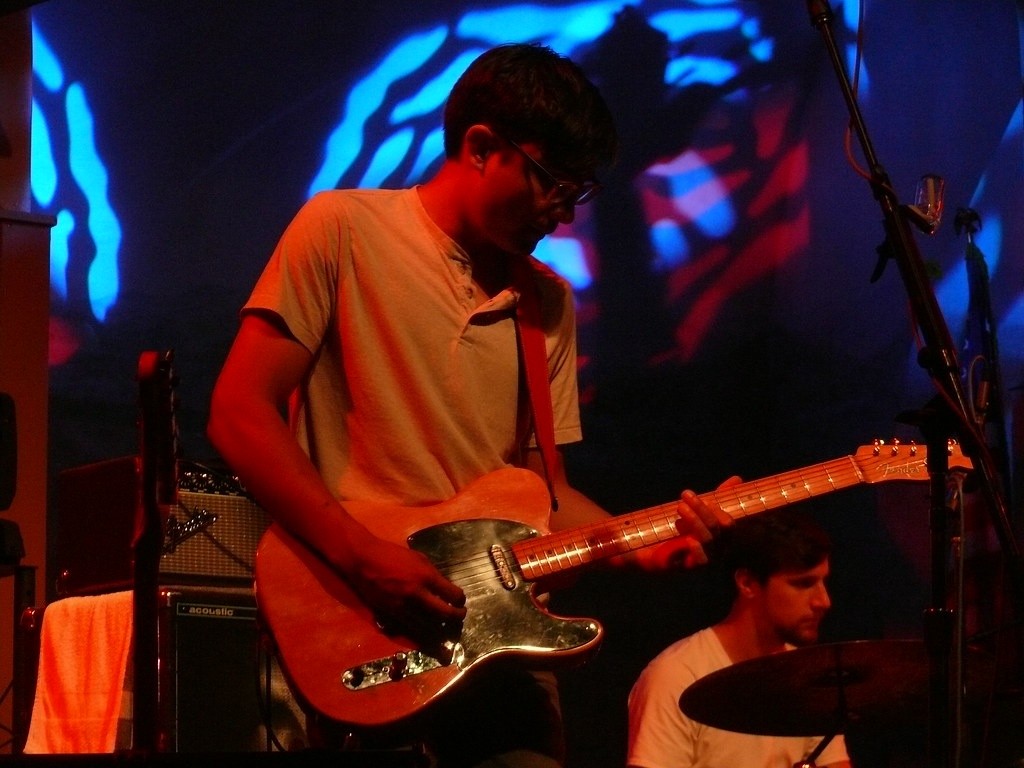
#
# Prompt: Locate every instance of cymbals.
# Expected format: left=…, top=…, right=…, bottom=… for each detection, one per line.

left=678, top=635, right=938, bottom=736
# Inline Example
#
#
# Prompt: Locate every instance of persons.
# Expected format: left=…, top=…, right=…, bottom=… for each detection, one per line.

left=623, top=512, right=853, bottom=768
left=205, top=40, right=743, bottom=768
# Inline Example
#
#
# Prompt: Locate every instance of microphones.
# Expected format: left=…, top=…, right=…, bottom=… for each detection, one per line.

left=913, top=174, right=946, bottom=234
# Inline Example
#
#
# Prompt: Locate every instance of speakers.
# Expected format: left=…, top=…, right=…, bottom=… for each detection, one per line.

left=14, top=456, right=328, bottom=760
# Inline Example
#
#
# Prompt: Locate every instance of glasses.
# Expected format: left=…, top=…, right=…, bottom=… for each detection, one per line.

left=464, top=119, right=606, bottom=206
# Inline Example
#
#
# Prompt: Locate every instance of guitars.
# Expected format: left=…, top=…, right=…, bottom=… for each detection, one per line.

left=254, top=435, right=977, bottom=731
